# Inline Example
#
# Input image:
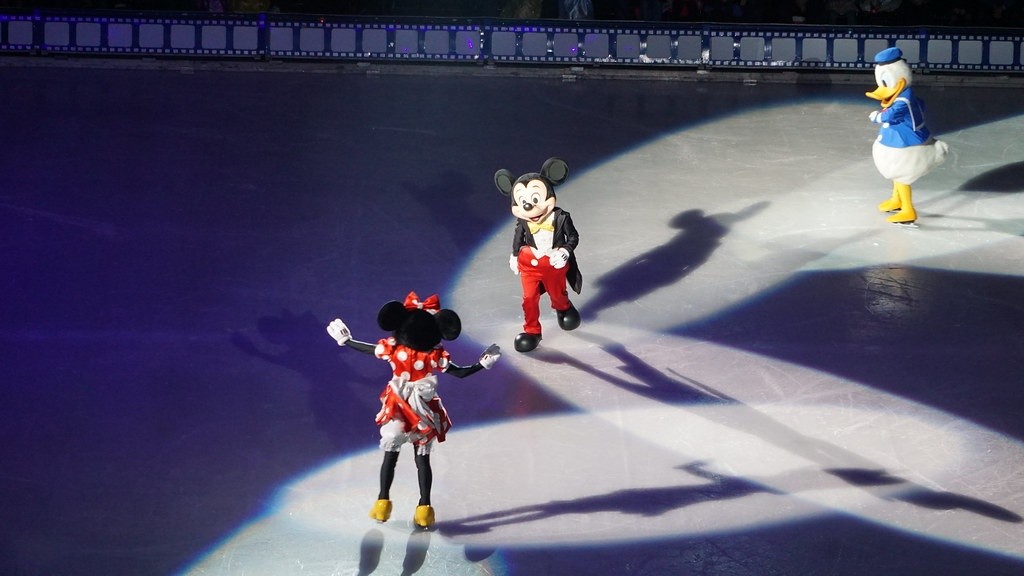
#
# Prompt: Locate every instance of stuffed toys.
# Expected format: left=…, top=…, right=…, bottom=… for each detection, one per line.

left=325, top=290, right=504, bottom=528
left=862, top=46, right=951, bottom=224
left=491, top=156, right=584, bottom=352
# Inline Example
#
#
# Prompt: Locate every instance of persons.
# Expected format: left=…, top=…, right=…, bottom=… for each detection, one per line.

left=496, top=1, right=1023, bottom=29
